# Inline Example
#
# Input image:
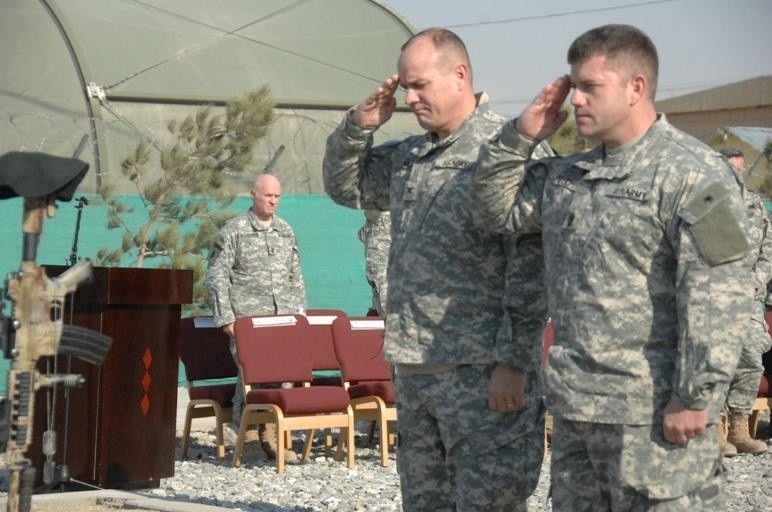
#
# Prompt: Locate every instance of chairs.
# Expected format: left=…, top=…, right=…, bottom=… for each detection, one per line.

left=176, top=309, right=397, bottom=474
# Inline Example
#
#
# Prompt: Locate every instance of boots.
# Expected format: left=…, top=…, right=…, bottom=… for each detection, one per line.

left=240, top=425, right=267, bottom=460
left=720, top=411, right=737, bottom=457
left=727, top=413, right=768, bottom=454
left=259, top=421, right=297, bottom=464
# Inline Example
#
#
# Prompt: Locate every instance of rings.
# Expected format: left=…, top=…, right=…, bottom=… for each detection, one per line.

left=506, top=402, right=516, bottom=409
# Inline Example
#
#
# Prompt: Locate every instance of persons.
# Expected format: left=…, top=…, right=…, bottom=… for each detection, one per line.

left=467, top=21, right=759, bottom=512
left=205, top=172, right=307, bottom=464
left=319, top=23, right=558, bottom=511
left=356, top=209, right=393, bottom=319
left=718, top=146, right=772, bottom=466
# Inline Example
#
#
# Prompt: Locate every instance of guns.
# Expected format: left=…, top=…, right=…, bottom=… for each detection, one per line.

left=1, top=192, right=113, bottom=512
